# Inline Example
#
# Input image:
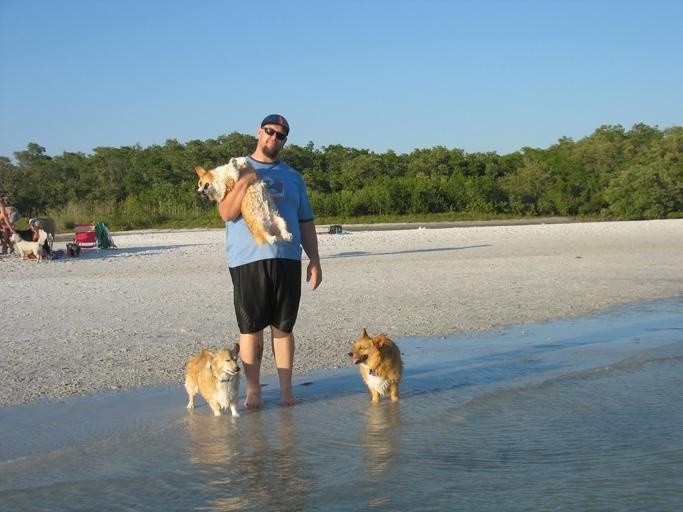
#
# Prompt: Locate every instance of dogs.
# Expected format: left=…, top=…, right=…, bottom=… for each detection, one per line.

left=8, top=229, right=48, bottom=263
left=182, top=340, right=244, bottom=418
left=346, top=326, right=404, bottom=404
left=189, top=157, right=295, bottom=246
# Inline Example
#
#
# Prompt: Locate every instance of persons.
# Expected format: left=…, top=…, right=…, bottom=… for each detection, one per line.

left=0, top=192, right=14, bottom=254
left=36, top=238, right=49, bottom=262
left=218, top=115, right=322, bottom=408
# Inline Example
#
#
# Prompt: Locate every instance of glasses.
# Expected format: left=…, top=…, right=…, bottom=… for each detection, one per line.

left=261, top=128, right=286, bottom=141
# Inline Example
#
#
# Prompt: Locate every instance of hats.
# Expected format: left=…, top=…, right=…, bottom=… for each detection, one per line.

left=261, top=114, right=290, bottom=136
